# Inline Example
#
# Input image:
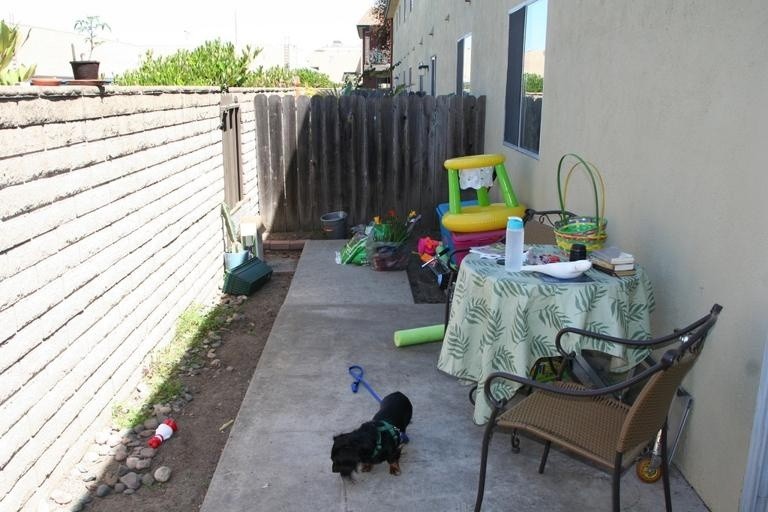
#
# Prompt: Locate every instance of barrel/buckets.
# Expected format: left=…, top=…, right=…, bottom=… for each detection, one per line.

left=224, top=249, right=249, bottom=272
left=320, top=210, right=348, bottom=240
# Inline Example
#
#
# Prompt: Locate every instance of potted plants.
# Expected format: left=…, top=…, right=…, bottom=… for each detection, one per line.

left=70, top=15, right=111, bottom=80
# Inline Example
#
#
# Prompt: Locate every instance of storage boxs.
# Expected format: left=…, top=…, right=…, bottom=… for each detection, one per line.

left=436, top=200, right=488, bottom=264
left=451, top=228, right=507, bottom=268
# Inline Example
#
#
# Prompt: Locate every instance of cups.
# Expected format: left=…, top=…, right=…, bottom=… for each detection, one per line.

left=569, top=243, right=586, bottom=262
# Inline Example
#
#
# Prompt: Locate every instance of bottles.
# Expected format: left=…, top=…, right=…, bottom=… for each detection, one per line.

left=505, top=216, right=525, bottom=273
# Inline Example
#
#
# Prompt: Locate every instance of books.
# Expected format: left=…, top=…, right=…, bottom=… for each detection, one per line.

left=592, top=246, right=637, bottom=276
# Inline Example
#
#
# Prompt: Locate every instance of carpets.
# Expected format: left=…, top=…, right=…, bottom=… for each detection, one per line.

left=406, top=249, right=452, bottom=303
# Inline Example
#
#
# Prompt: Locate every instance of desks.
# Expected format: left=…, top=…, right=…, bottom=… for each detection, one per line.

left=436, top=243, right=656, bottom=426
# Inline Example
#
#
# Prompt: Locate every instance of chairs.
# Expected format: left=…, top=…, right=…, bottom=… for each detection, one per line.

left=444, top=208, right=579, bottom=337
left=475, top=303, right=722, bottom=512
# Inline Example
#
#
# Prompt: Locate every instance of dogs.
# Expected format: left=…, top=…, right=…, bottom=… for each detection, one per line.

left=330, top=391, right=413, bottom=485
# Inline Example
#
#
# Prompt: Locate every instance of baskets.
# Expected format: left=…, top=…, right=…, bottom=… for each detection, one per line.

left=552, top=161, right=608, bottom=257
left=554, top=154, right=607, bottom=235
